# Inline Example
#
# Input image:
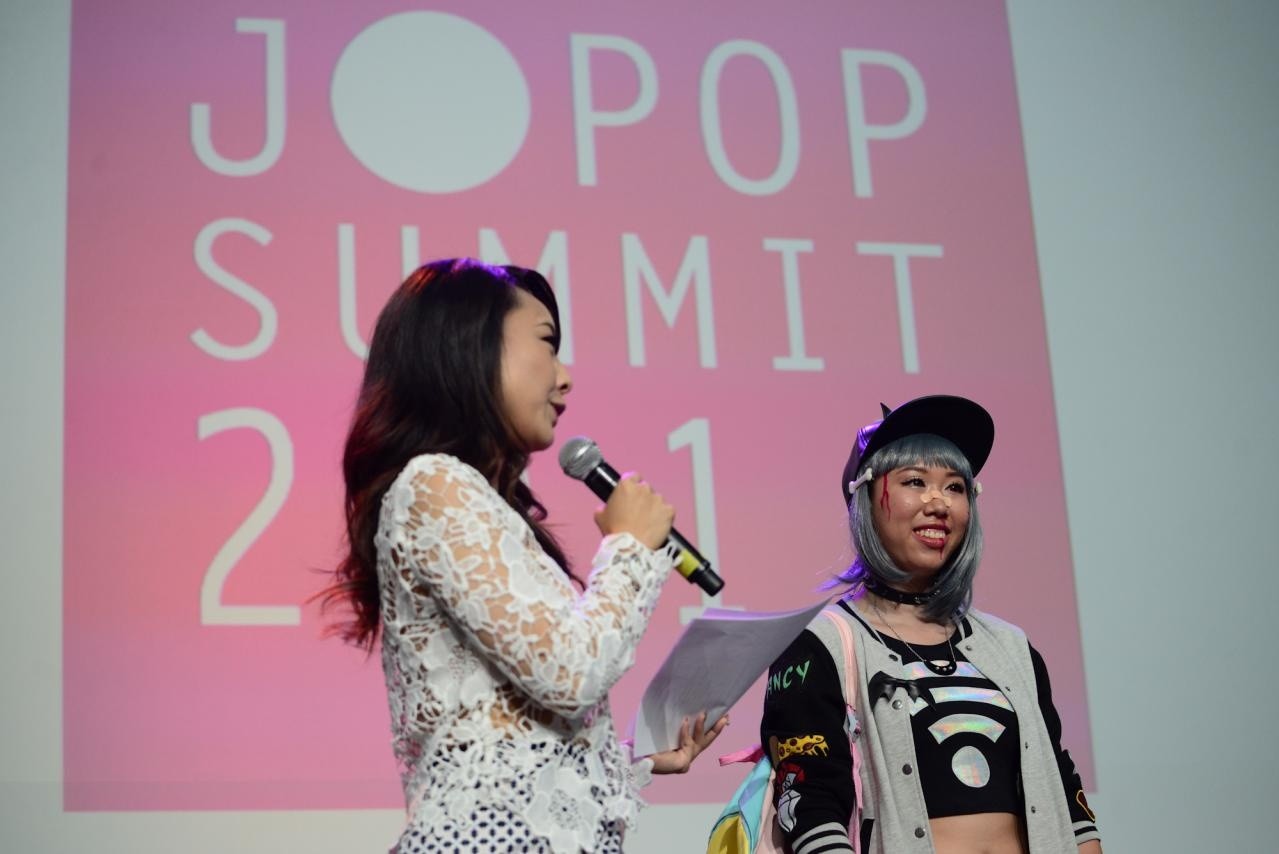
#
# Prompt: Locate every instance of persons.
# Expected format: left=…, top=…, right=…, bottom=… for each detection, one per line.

left=761, top=395, right=1105, bottom=854
left=319, top=256, right=729, bottom=854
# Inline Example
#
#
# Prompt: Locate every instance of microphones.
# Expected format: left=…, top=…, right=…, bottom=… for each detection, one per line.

left=558, top=436, right=724, bottom=598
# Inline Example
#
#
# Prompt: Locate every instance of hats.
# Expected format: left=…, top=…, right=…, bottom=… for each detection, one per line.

left=843, top=394, right=995, bottom=508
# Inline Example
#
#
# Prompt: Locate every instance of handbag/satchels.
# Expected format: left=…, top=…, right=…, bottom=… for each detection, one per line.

left=706, top=611, right=867, bottom=854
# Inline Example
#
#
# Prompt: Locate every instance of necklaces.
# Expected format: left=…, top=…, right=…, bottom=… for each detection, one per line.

left=874, top=608, right=957, bottom=671
